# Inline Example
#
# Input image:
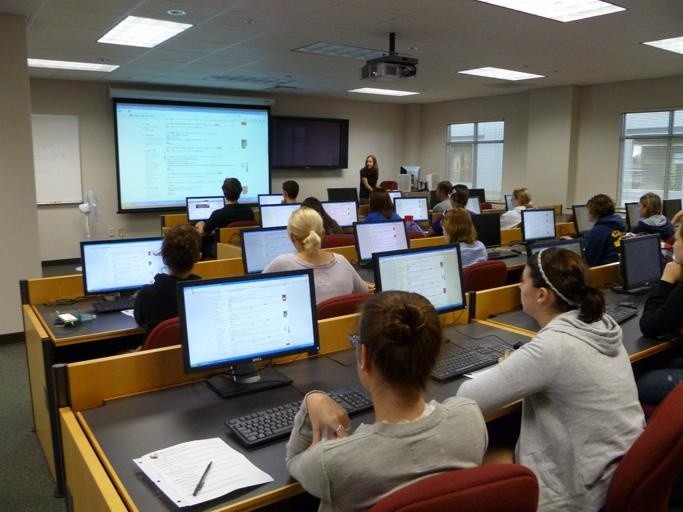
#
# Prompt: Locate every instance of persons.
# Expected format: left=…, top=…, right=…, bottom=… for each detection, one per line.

left=442, top=208, right=487, bottom=267
left=630, top=192, right=675, bottom=242
left=381, top=180, right=398, bottom=190
left=134, top=224, right=202, bottom=350
left=285, top=290, right=489, bottom=511
left=639, top=209, right=683, bottom=406
left=301, top=197, right=342, bottom=234
left=281, top=180, right=299, bottom=204
left=359, top=155, right=378, bottom=205
left=561, top=194, right=625, bottom=267
left=428, top=181, right=475, bottom=237
left=264, top=207, right=368, bottom=303
left=457, top=247, right=648, bottom=512
left=195, top=178, right=255, bottom=261
left=365, top=188, right=401, bottom=223
left=500, top=188, right=533, bottom=229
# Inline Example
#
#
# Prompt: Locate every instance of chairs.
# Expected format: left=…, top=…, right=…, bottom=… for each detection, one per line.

left=129, top=182, right=683, bottom=512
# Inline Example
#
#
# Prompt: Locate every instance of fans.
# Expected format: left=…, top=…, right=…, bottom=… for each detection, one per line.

left=78, top=190, right=101, bottom=238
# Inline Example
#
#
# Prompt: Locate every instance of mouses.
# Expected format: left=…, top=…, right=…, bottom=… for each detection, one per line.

left=513, top=342, right=523, bottom=349
left=617, top=301, right=637, bottom=309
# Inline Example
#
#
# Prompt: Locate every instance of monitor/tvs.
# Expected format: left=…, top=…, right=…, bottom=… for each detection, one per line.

left=186, top=196, right=226, bottom=222
left=241, top=226, right=298, bottom=273
left=327, top=188, right=360, bottom=208
left=504, top=195, right=513, bottom=211
left=387, top=191, right=402, bottom=204
left=258, top=194, right=284, bottom=206
left=320, top=201, right=357, bottom=227
left=431, top=190, right=442, bottom=210
left=572, top=204, right=595, bottom=233
left=521, top=208, right=556, bottom=244
left=527, top=240, right=585, bottom=267
left=353, top=219, right=410, bottom=262
left=372, top=244, right=467, bottom=343
left=471, top=213, right=501, bottom=248
left=80, top=237, right=172, bottom=302
left=616, top=233, right=662, bottom=295
left=393, top=196, right=429, bottom=222
left=176, top=268, right=320, bottom=400
left=259, top=202, right=302, bottom=228
left=467, top=188, right=486, bottom=203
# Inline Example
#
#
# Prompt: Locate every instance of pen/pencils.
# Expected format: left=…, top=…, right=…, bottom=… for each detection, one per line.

left=193, top=460, right=212, bottom=497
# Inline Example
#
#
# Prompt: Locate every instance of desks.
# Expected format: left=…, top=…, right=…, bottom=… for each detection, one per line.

left=51, top=292, right=537, bottom=511
left=19, top=245, right=376, bottom=499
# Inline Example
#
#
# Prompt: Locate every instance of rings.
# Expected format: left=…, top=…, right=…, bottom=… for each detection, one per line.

left=335, top=424, right=345, bottom=434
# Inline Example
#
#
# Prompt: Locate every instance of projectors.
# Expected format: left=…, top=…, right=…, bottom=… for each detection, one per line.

left=359, top=63, right=417, bottom=82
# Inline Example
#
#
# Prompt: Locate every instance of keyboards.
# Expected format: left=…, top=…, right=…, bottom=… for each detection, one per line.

left=225, top=386, right=374, bottom=449
left=92, top=299, right=136, bottom=312
left=428, top=335, right=517, bottom=383
left=488, top=250, right=518, bottom=259
left=604, top=304, right=639, bottom=324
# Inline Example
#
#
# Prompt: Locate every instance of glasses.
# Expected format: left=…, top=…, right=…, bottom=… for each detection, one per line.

left=347, top=333, right=363, bottom=348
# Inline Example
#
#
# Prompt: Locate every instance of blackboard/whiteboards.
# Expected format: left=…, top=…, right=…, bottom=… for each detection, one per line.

left=31, top=114, right=83, bottom=206
